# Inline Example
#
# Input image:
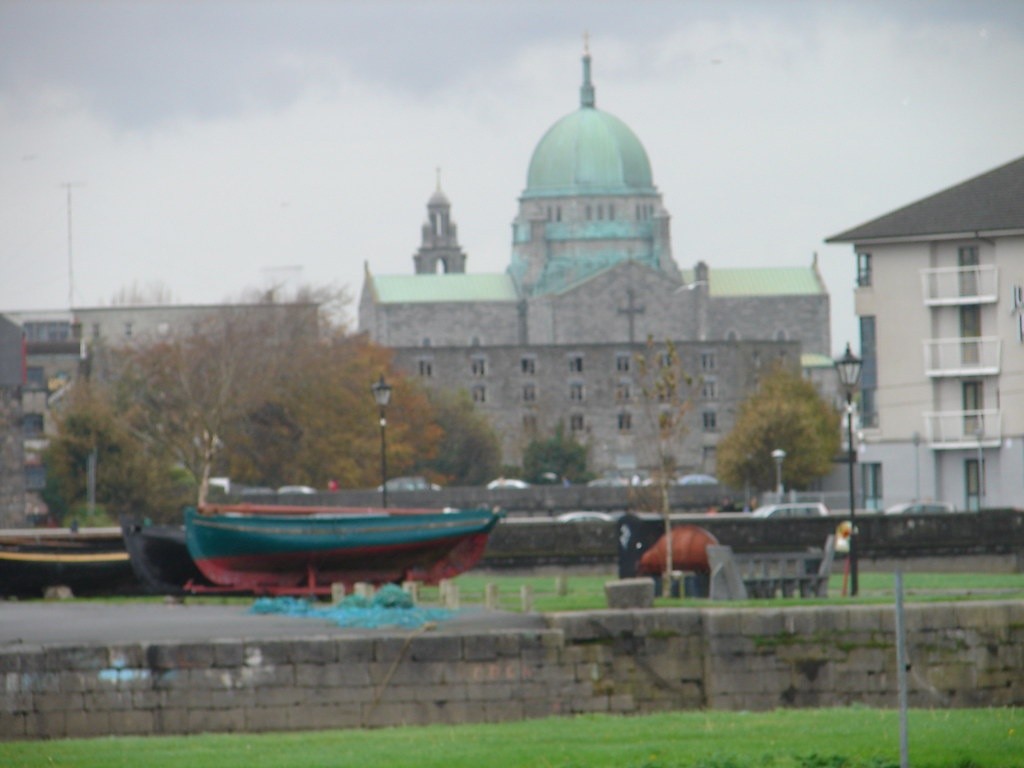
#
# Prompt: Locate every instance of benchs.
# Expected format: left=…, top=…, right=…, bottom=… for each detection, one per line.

left=711, top=550, right=829, bottom=599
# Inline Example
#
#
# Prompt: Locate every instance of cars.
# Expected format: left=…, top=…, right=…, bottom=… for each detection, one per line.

left=876, top=498, right=958, bottom=514
left=749, top=503, right=830, bottom=518
left=208, top=473, right=723, bottom=495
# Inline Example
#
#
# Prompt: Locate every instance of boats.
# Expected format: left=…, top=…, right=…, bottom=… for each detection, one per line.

left=1, top=534, right=129, bottom=595
left=178, top=504, right=504, bottom=594
left=123, top=515, right=194, bottom=595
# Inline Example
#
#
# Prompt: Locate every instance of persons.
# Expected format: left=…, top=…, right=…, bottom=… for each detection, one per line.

left=617, top=506, right=643, bottom=580
left=718, top=495, right=737, bottom=513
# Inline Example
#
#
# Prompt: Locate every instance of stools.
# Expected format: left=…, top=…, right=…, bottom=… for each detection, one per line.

left=604, top=577, right=654, bottom=610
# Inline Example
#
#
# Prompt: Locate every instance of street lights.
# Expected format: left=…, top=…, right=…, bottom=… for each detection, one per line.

left=773, top=447, right=785, bottom=504
left=371, top=371, right=393, bottom=508
left=832, top=340, right=864, bottom=596
